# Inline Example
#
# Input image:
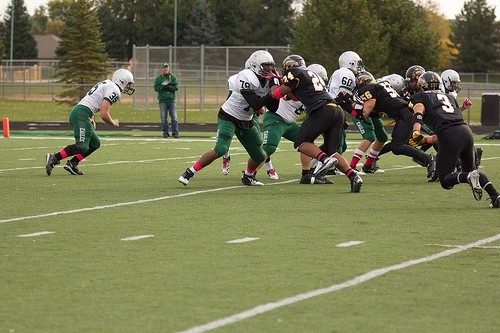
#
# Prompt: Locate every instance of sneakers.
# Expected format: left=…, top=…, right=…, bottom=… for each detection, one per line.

left=177, top=167, right=194, bottom=185
left=427, top=151, right=437, bottom=177
left=45, top=153, right=60, bottom=176
left=64, top=159, right=84, bottom=176
left=474, top=147, right=483, bottom=168
left=486, top=192, right=500, bottom=208
left=469, top=169, right=482, bottom=201
left=222, top=155, right=230, bottom=175
left=267, top=169, right=279, bottom=179
left=240, top=170, right=264, bottom=186
left=300, top=155, right=385, bottom=192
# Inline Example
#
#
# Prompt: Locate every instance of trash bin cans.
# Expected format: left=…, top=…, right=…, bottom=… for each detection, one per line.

left=480, top=92, right=500, bottom=125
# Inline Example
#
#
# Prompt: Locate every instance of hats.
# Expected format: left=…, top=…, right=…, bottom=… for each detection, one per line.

left=161, top=63, right=169, bottom=68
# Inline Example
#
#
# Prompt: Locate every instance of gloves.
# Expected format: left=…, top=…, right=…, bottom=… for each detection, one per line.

left=408, top=130, right=427, bottom=147
left=341, top=91, right=353, bottom=105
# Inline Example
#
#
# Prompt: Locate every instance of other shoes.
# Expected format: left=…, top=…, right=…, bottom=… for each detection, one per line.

left=174, top=133, right=179, bottom=138
left=163, top=133, right=168, bottom=138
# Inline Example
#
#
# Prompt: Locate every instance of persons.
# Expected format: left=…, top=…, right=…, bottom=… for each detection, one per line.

left=179, top=50, right=500, bottom=210
left=154, top=63, right=179, bottom=138
left=45, top=69, right=135, bottom=176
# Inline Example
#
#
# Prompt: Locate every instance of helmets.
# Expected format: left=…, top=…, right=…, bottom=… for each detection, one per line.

left=355, top=69, right=375, bottom=88
left=338, top=51, right=365, bottom=76
left=244, top=49, right=276, bottom=80
left=405, top=66, right=425, bottom=94
left=112, top=68, right=136, bottom=96
left=282, top=54, right=306, bottom=72
left=416, top=70, right=441, bottom=92
left=440, top=69, right=460, bottom=92
left=306, top=64, right=328, bottom=83
left=382, top=73, right=406, bottom=95
